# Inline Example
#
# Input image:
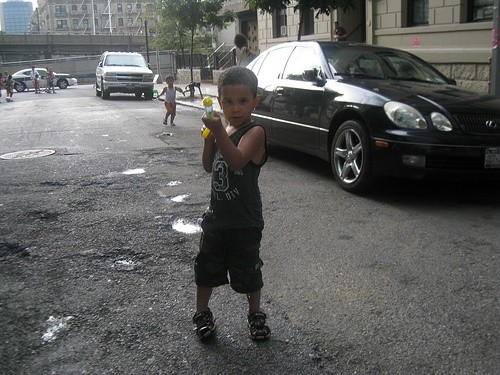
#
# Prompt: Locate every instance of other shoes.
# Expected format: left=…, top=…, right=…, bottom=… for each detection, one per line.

left=35, top=91, right=42, bottom=94
left=46, top=90, right=51, bottom=94
left=53, top=92, right=57, bottom=94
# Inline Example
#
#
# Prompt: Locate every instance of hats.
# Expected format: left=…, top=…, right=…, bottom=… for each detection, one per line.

left=30, top=66, right=35, bottom=68
left=49, top=67, right=53, bottom=71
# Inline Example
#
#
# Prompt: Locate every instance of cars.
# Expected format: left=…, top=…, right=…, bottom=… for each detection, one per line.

left=245, top=38, right=500, bottom=192
left=11, top=68, right=78, bottom=92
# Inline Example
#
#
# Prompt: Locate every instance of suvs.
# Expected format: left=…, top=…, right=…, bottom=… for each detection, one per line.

left=95, top=50, right=155, bottom=100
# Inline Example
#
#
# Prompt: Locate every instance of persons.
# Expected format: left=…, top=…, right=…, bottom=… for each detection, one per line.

left=333, top=21, right=347, bottom=41
left=182, top=81, right=204, bottom=99
left=30, top=66, right=57, bottom=94
left=193, top=66, right=272, bottom=341
left=0, top=71, right=15, bottom=103
left=157, top=76, right=182, bottom=126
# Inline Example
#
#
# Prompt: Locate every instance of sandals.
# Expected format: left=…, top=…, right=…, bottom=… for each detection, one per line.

left=192, top=306, right=217, bottom=341
left=163, top=119, right=168, bottom=126
left=170, top=122, right=176, bottom=126
left=246, top=310, right=271, bottom=340
